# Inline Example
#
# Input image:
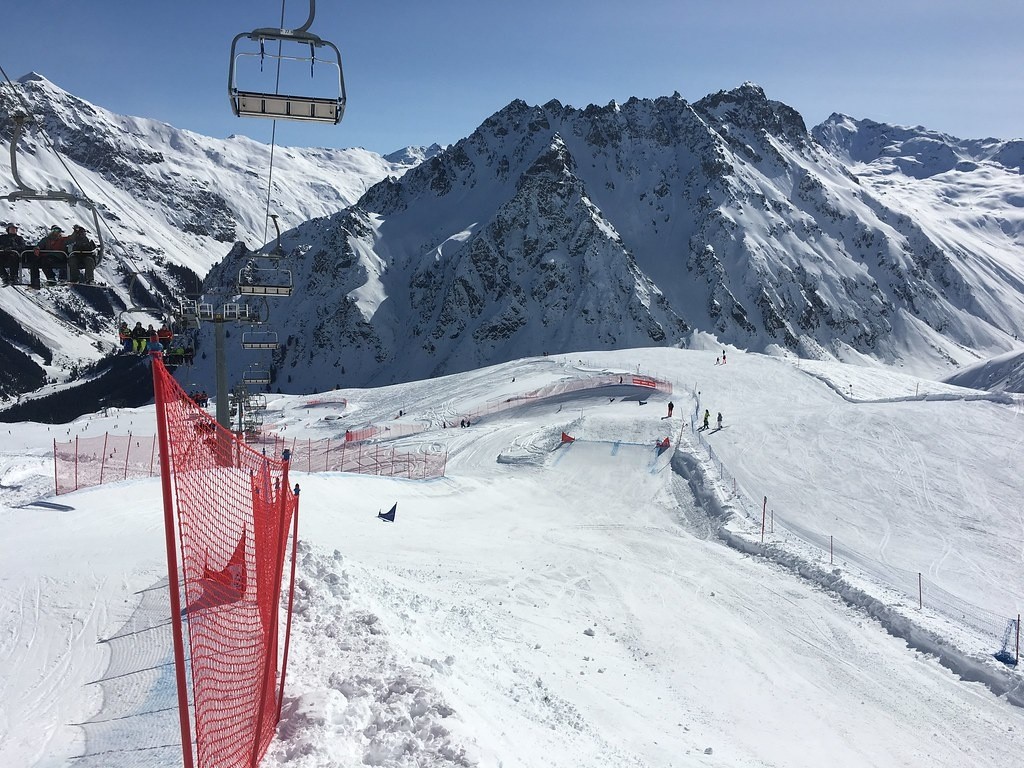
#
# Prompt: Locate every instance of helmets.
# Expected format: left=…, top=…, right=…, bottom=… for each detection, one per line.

left=51, top=225, right=62, bottom=233
left=6, top=223, right=18, bottom=232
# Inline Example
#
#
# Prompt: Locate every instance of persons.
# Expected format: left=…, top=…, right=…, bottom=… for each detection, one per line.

left=194, top=419, right=215, bottom=432
left=162, top=343, right=193, bottom=365
left=718, top=412, right=723, bottom=428
left=119, top=322, right=173, bottom=356
left=180, top=390, right=207, bottom=408
left=668, top=401, right=674, bottom=417
left=461, top=419, right=470, bottom=428
left=716, top=350, right=726, bottom=364
left=0, top=223, right=95, bottom=288
left=703, top=409, right=710, bottom=428
